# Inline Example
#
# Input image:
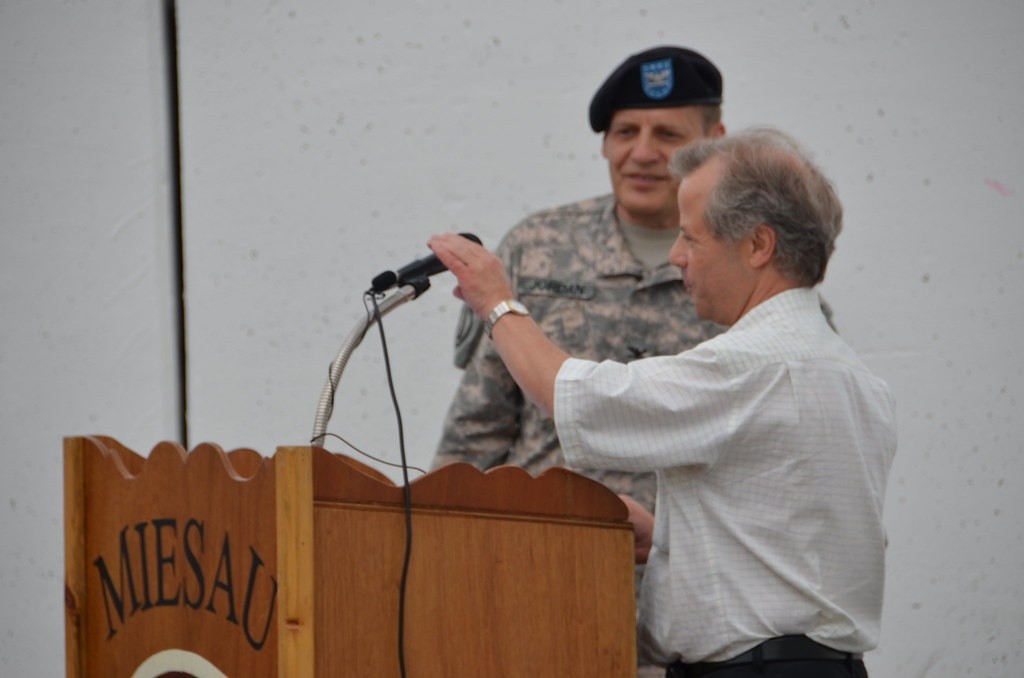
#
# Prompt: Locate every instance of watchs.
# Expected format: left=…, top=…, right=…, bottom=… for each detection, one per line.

left=485, top=299, right=529, bottom=340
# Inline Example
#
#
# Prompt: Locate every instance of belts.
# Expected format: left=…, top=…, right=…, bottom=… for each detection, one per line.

left=667, top=632, right=861, bottom=678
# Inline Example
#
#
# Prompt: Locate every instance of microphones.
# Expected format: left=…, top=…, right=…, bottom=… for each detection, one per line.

left=372, top=233, right=483, bottom=294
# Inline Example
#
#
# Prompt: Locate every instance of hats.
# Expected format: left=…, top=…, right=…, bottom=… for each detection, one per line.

left=588, top=47, right=725, bottom=132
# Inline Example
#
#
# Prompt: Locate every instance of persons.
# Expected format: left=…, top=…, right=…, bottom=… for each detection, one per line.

left=423, top=125, right=899, bottom=678
left=428, top=48, right=842, bottom=667
